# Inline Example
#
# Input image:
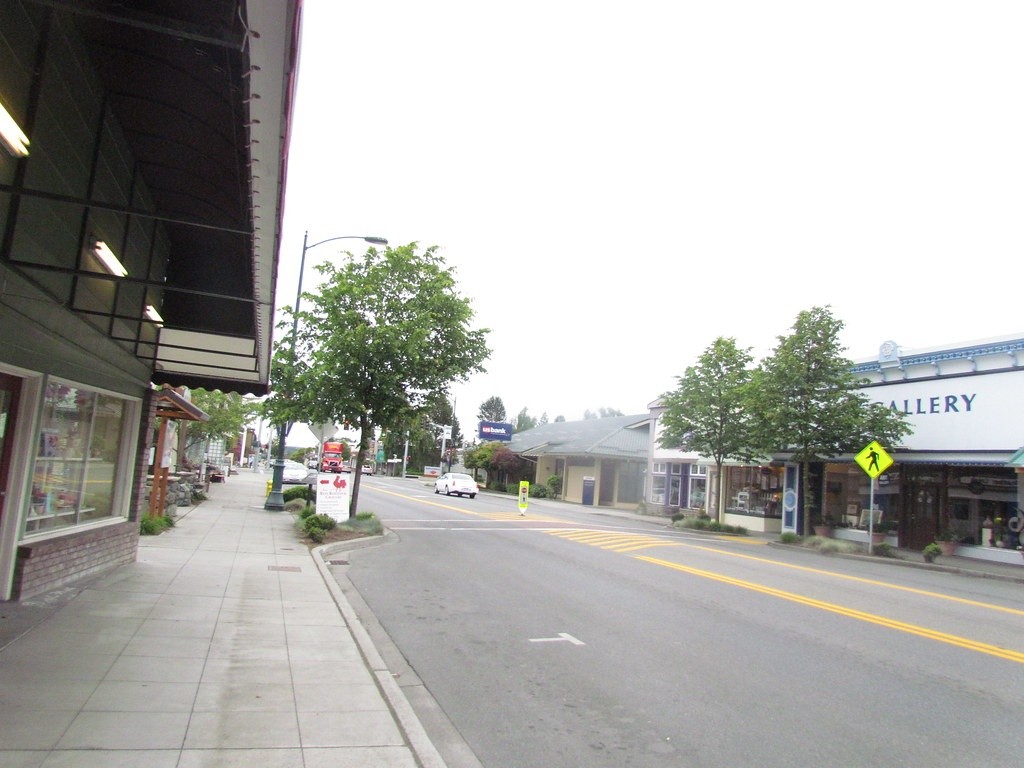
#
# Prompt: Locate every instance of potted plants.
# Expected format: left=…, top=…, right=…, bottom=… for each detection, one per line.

left=810, top=515, right=833, bottom=539
left=933, top=528, right=956, bottom=557
left=867, top=522, right=889, bottom=543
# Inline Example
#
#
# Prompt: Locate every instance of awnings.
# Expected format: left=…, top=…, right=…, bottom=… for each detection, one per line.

left=698, top=449, right=1024, bottom=467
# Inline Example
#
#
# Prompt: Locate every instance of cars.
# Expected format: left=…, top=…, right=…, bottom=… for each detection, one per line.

left=434, top=473, right=479, bottom=499
left=341, top=461, right=352, bottom=473
left=249, top=453, right=320, bottom=486
left=361, top=464, right=373, bottom=476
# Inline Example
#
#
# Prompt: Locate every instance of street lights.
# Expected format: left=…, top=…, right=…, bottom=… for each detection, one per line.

left=263, top=231, right=388, bottom=513
left=445, top=395, right=457, bottom=473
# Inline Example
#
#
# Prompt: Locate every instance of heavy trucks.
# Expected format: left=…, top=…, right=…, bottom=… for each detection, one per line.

left=321, top=442, right=344, bottom=473
